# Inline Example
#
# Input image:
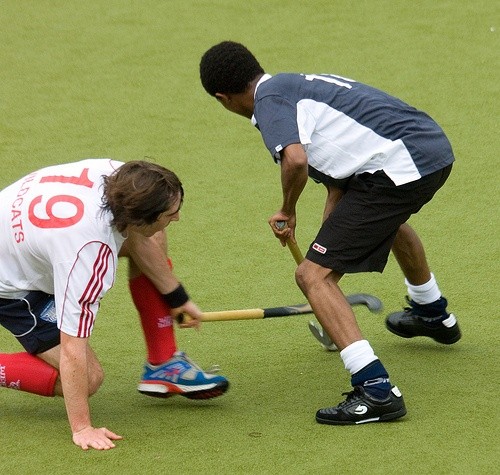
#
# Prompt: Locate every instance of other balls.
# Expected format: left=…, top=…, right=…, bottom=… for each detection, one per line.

left=321, top=341, right=339, bottom=351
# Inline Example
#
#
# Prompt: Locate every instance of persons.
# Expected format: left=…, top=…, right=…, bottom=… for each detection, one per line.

left=1, top=159, right=228, bottom=450
left=201, top=42, right=462, bottom=423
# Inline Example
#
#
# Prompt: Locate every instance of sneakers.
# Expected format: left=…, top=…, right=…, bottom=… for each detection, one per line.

left=386, top=294, right=461, bottom=344
left=316, top=384, right=407, bottom=425
left=137, top=351, right=228, bottom=399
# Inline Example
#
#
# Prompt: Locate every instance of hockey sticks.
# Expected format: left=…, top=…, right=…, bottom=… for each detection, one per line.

left=273, top=222, right=336, bottom=351
left=173, top=292, right=384, bottom=325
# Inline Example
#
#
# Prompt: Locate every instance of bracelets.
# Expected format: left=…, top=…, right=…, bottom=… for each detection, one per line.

left=161, top=284, right=189, bottom=308
